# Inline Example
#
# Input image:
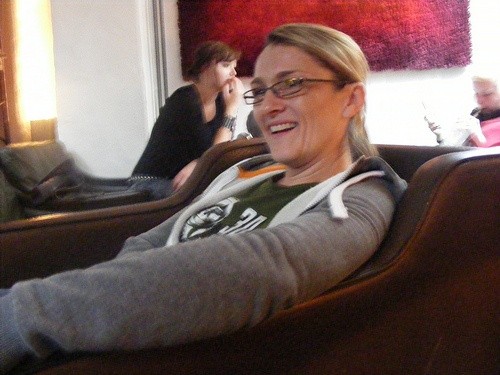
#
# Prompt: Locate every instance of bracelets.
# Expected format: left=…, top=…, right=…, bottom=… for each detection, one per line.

left=217, top=113, right=237, bottom=139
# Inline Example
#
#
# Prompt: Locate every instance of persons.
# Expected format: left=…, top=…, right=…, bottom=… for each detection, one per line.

left=424, top=74, right=500, bottom=146
left=0, top=23, right=408, bottom=369
left=126, top=41, right=245, bottom=198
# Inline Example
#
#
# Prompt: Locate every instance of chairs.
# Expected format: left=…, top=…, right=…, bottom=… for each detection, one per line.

left=0, top=136, right=500, bottom=375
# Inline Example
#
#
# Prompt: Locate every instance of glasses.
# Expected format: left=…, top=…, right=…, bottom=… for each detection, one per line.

left=243, top=76, right=340, bottom=105
